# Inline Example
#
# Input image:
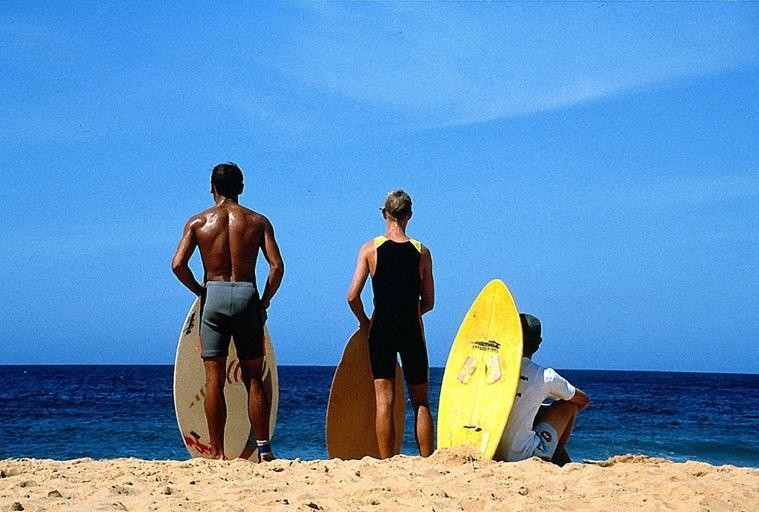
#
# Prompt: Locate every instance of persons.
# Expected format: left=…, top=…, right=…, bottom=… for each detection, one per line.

left=346, top=189, right=433, bottom=458
left=494, top=312, right=591, bottom=468
left=171, top=163, right=284, bottom=462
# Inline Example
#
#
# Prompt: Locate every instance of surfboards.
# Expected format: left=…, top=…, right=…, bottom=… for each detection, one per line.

left=436, top=278, right=524, bottom=461
left=325, top=326, right=405, bottom=459
left=173, top=295, right=279, bottom=460
